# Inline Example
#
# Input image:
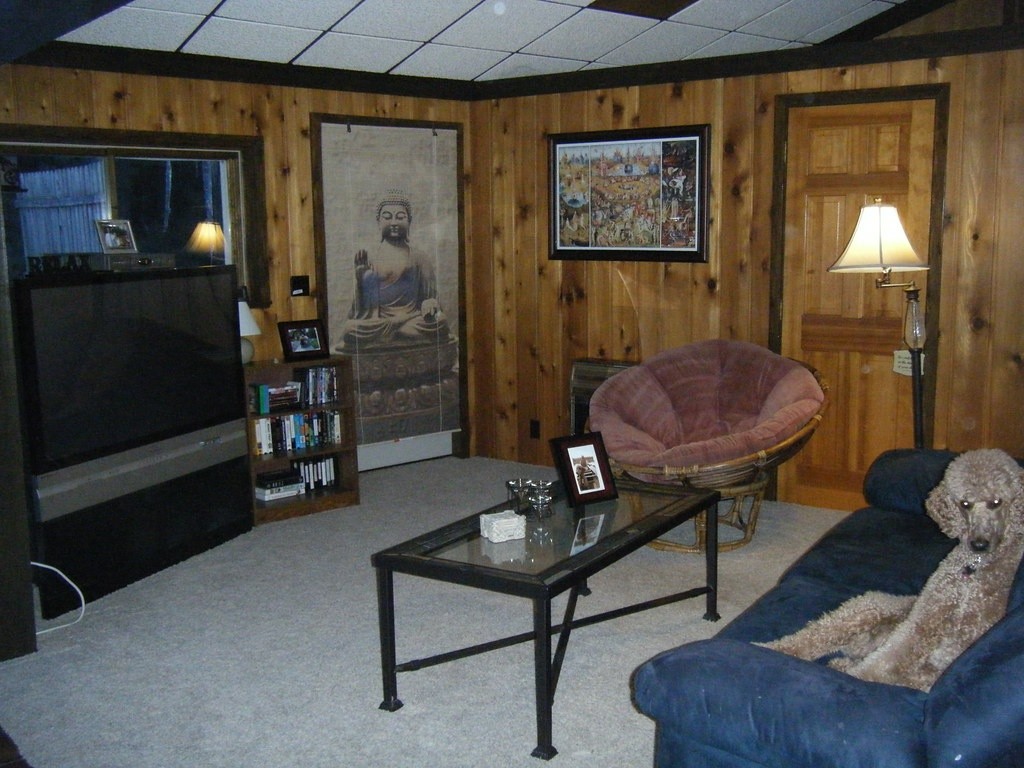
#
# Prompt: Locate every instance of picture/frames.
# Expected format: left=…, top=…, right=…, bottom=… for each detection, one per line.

left=547, top=123, right=711, bottom=263
left=309, top=110, right=468, bottom=444
left=277, top=320, right=330, bottom=363
left=549, top=432, right=619, bottom=507
left=96, top=219, right=138, bottom=254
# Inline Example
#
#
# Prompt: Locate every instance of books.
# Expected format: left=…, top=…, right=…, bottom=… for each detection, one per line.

left=250, top=366, right=341, bottom=501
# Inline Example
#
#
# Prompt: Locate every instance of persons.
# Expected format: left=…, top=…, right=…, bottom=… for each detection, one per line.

left=105, top=227, right=124, bottom=247
left=343, top=187, right=450, bottom=343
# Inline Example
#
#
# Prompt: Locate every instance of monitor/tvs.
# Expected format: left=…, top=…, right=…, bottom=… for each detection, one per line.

left=9, top=263, right=249, bottom=523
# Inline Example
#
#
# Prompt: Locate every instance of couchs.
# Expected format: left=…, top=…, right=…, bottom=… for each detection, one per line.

left=628, top=450, right=1024, bottom=768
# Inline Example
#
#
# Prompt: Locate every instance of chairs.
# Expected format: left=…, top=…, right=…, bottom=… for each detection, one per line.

left=584, top=338, right=829, bottom=555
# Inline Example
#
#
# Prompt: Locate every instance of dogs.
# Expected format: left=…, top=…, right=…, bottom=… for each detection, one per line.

left=749, top=447, right=1024, bottom=695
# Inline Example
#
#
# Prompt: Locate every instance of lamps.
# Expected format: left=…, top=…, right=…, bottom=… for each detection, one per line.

left=181, top=219, right=226, bottom=258
left=826, top=198, right=931, bottom=449
left=238, top=302, right=262, bottom=363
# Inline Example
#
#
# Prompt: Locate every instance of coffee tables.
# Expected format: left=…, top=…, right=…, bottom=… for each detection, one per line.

left=371, top=480, right=722, bottom=763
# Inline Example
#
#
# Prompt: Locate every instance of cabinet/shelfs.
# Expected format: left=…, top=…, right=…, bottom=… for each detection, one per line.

left=243, top=353, right=362, bottom=525
